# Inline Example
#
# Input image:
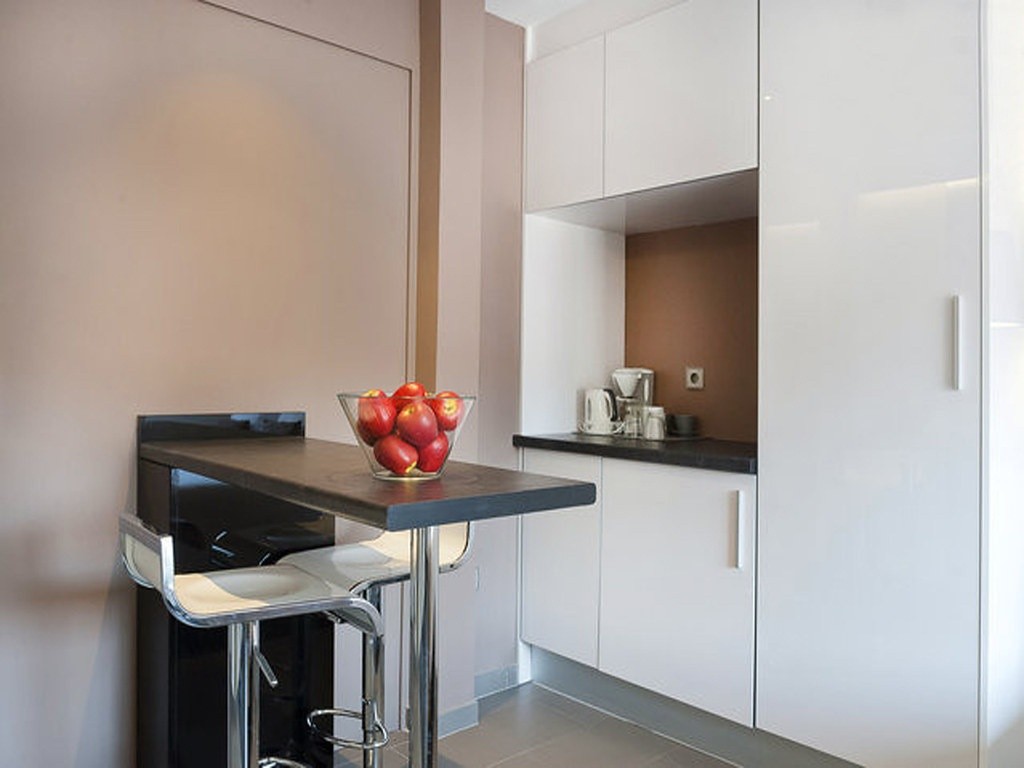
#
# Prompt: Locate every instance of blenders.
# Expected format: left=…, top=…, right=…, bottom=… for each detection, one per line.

left=613, top=369, right=643, bottom=438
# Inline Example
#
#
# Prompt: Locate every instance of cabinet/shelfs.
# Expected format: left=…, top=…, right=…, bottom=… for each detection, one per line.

left=754, top=0, right=1024, bottom=768
left=520, top=449, right=757, bottom=733
left=523, top=0, right=758, bottom=237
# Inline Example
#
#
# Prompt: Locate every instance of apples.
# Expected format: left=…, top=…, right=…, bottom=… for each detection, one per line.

left=354, top=381, right=465, bottom=477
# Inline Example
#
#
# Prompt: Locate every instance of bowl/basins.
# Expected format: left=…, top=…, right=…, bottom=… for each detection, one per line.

left=337, top=391, right=475, bottom=481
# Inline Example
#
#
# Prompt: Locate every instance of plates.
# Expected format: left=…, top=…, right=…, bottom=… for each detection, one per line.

left=669, top=429, right=701, bottom=437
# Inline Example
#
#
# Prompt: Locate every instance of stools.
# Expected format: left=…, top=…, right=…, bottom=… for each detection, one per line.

left=277, top=521, right=475, bottom=767
left=117, top=512, right=389, bottom=767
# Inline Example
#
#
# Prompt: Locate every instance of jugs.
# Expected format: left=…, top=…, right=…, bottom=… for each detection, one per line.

left=585, top=389, right=612, bottom=431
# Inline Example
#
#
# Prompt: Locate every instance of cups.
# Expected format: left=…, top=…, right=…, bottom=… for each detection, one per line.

left=671, top=415, right=695, bottom=430
left=646, top=414, right=665, bottom=439
